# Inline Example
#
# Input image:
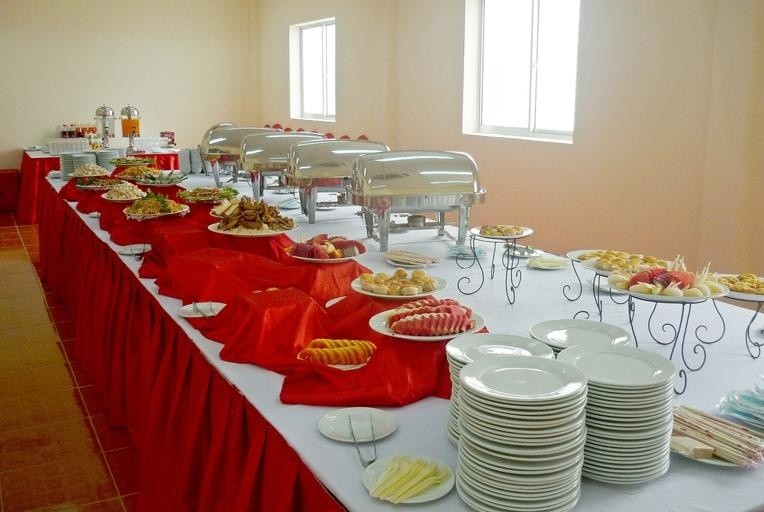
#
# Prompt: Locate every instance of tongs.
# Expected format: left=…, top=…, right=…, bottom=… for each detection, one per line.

left=346, top=414, right=378, bottom=465
left=129, top=245, right=146, bottom=260
left=192, top=303, right=216, bottom=320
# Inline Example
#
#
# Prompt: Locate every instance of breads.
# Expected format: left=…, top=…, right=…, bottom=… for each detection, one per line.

left=578, top=250, right=605, bottom=260
left=479, top=224, right=523, bottom=238
left=595, top=251, right=668, bottom=272
left=358, top=267, right=438, bottom=296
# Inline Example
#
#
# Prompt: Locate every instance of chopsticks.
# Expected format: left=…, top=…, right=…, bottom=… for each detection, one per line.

left=672, top=404, right=763, bottom=470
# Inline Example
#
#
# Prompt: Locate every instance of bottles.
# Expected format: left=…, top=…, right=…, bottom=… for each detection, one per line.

left=61, top=122, right=97, bottom=138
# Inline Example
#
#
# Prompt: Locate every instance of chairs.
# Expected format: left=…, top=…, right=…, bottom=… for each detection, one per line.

left=260, top=122, right=370, bottom=191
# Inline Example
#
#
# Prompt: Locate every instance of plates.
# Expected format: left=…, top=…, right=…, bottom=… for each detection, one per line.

left=59, top=147, right=151, bottom=180
left=529, top=319, right=630, bottom=358
left=471, top=227, right=533, bottom=239
left=294, top=349, right=370, bottom=373
left=288, top=242, right=365, bottom=261
left=559, top=344, right=678, bottom=487
left=209, top=208, right=282, bottom=222
left=361, top=455, right=454, bottom=504
left=443, top=334, right=555, bottom=451
left=124, top=204, right=189, bottom=218
left=454, top=357, right=585, bottom=510
left=349, top=273, right=449, bottom=297
left=567, top=249, right=608, bottom=265
left=206, top=218, right=297, bottom=237
left=135, top=181, right=180, bottom=186
left=177, top=302, right=226, bottom=317
left=118, top=243, right=151, bottom=254
left=682, top=452, right=741, bottom=469
left=317, top=406, right=397, bottom=442
left=23, top=146, right=41, bottom=151
left=369, top=309, right=485, bottom=341
left=714, top=273, right=764, bottom=304
left=388, top=259, right=431, bottom=268
left=610, top=272, right=726, bottom=302
left=524, top=257, right=566, bottom=270
left=578, top=260, right=678, bottom=275
left=100, top=193, right=148, bottom=201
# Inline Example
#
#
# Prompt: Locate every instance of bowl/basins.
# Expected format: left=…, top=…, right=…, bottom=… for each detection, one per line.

left=177, top=147, right=213, bottom=174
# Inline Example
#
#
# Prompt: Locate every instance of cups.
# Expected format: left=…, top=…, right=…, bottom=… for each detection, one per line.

left=129, top=131, right=176, bottom=153
left=91, top=135, right=103, bottom=150
left=47, top=137, right=89, bottom=156
